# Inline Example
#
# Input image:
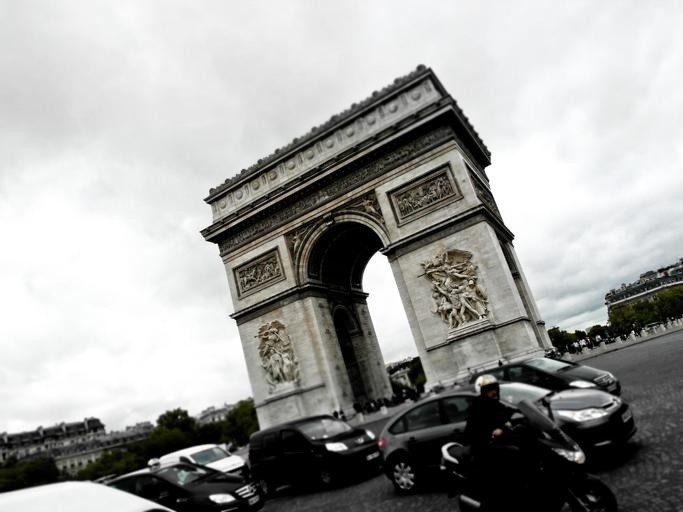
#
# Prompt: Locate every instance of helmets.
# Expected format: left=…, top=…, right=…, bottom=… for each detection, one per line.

left=474, top=374, right=498, bottom=396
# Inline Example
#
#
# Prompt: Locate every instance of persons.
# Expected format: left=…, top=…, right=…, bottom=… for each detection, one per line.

left=332, top=381, right=467, bottom=423
left=544, top=349, right=557, bottom=359
left=564, top=319, right=643, bottom=354
left=420, top=249, right=489, bottom=328
left=497, top=359, right=502, bottom=367
left=467, top=367, right=472, bottom=374
left=257, top=324, right=293, bottom=386
left=463, top=373, right=523, bottom=479
